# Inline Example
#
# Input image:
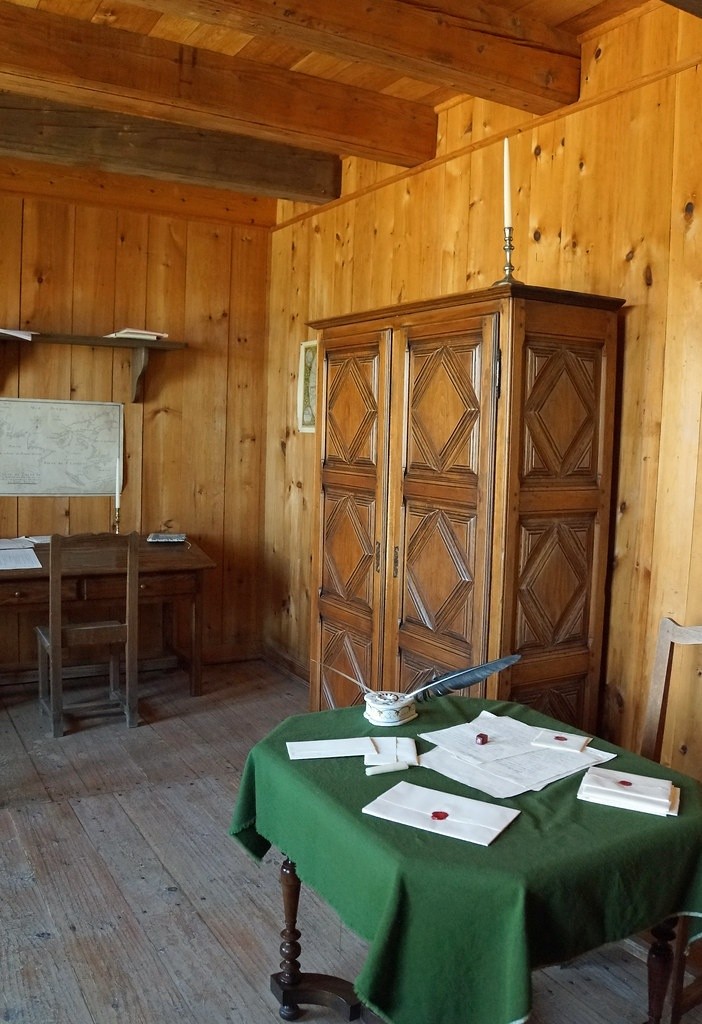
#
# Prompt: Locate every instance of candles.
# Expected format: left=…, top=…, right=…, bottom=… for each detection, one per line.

left=503, top=136, right=514, bottom=232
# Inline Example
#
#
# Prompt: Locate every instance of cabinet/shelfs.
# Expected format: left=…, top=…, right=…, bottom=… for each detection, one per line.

left=307, top=282, right=628, bottom=739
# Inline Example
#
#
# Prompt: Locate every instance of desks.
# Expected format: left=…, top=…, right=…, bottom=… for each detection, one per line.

left=230, top=695, right=702, bottom=1024
left=0, top=533, right=217, bottom=698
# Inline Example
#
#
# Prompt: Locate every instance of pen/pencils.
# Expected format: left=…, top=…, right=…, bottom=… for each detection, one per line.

left=368, top=735, right=381, bottom=755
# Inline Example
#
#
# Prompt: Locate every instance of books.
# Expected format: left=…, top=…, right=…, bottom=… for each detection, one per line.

left=0, top=326, right=40, bottom=342
left=104, top=327, right=170, bottom=341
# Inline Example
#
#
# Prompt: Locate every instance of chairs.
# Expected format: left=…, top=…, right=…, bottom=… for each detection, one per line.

left=35, top=532, right=140, bottom=737
left=613, top=616, right=702, bottom=1024
